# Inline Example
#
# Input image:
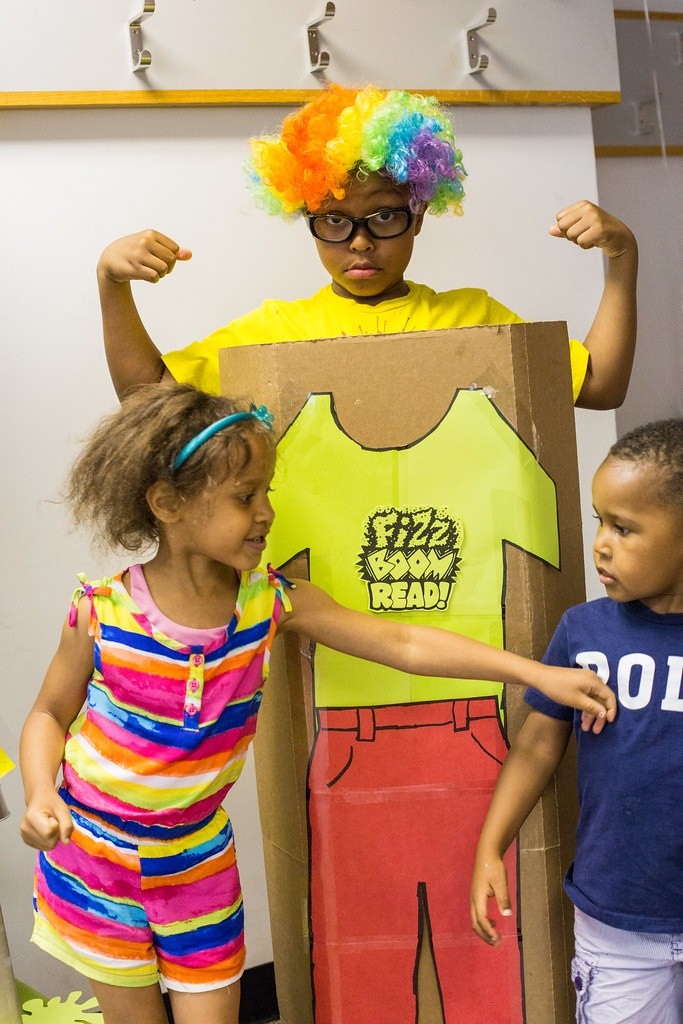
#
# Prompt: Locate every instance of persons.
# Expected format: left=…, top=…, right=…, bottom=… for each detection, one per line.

left=93, top=88, right=639, bottom=428
left=14, top=381, right=623, bottom=1024
left=469, top=415, right=679, bottom=1023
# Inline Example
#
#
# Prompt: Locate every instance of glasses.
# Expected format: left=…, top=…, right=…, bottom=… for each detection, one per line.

left=299, top=201, right=421, bottom=243
